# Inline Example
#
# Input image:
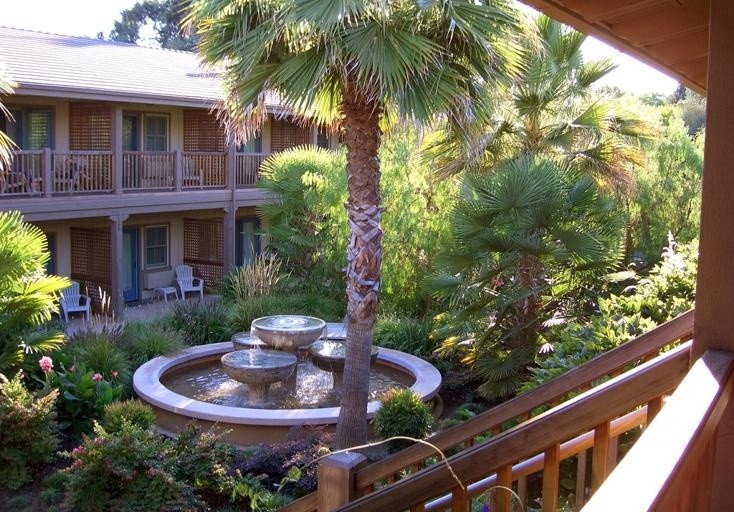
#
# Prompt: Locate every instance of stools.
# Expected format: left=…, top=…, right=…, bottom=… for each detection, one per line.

left=152, top=286, right=179, bottom=304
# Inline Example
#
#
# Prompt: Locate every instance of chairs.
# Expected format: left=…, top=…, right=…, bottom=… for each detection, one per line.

left=53, top=279, right=93, bottom=326
left=174, top=263, right=204, bottom=299
